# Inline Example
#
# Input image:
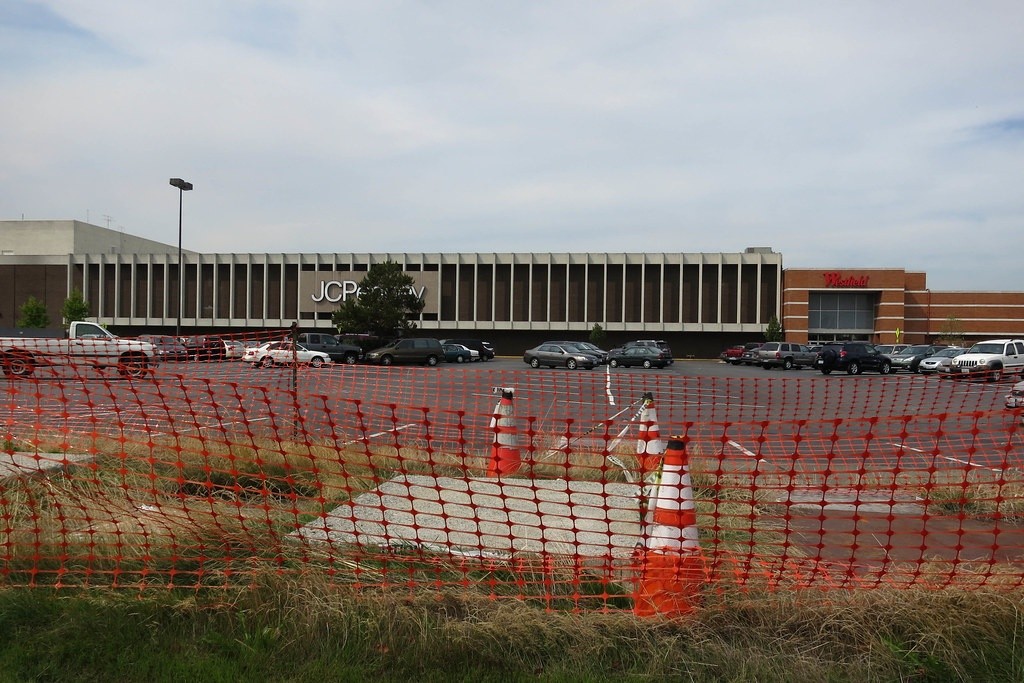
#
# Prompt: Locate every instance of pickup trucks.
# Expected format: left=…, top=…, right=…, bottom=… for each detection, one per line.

left=281, top=333, right=363, bottom=365
left=0, top=320, right=162, bottom=379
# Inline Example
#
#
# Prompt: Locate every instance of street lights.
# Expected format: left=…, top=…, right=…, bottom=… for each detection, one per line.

left=169, top=178, right=194, bottom=343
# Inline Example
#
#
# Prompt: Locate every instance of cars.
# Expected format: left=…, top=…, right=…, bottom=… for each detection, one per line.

left=1005, top=381, right=1024, bottom=417
left=868, top=343, right=914, bottom=361
left=221, top=339, right=266, bottom=359
left=605, top=346, right=666, bottom=369
left=241, top=341, right=331, bottom=368
left=438, top=338, right=496, bottom=363
left=137, top=335, right=188, bottom=361
left=720, top=340, right=880, bottom=367
left=543, top=341, right=608, bottom=366
left=523, top=343, right=600, bottom=370
left=185, top=335, right=227, bottom=362
left=366, top=338, right=446, bottom=366
left=917, top=347, right=979, bottom=376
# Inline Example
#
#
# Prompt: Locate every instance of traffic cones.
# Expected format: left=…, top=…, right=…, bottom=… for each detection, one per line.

left=634, top=392, right=664, bottom=473
left=632, top=438, right=708, bottom=616
left=486, top=389, right=521, bottom=478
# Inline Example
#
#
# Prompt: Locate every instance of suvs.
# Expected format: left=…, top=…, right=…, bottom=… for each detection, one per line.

left=758, top=342, right=815, bottom=370
left=610, top=340, right=674, bottom=366
left=891, top=343, right=952, bottom=373
left=949, top=338, right=1024, bottom=383
left=817, top=341, right=893, bottom=375
